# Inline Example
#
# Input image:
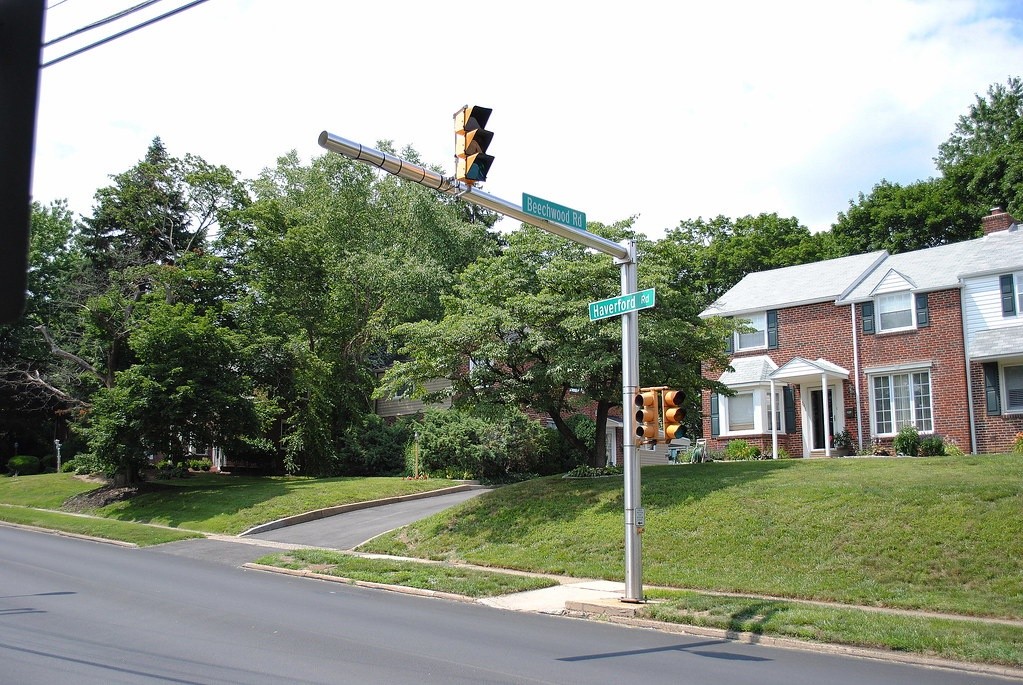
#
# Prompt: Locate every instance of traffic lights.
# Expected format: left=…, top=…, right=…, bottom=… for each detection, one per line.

left=634, top=390, right=659, bottom=440
left=453, top=104, right=494, bottom=185
left=661, top=389, right=688, bottom=443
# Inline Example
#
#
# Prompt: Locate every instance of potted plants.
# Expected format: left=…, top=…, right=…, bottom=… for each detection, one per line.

left=835, top=429, right=851, bottom=457
